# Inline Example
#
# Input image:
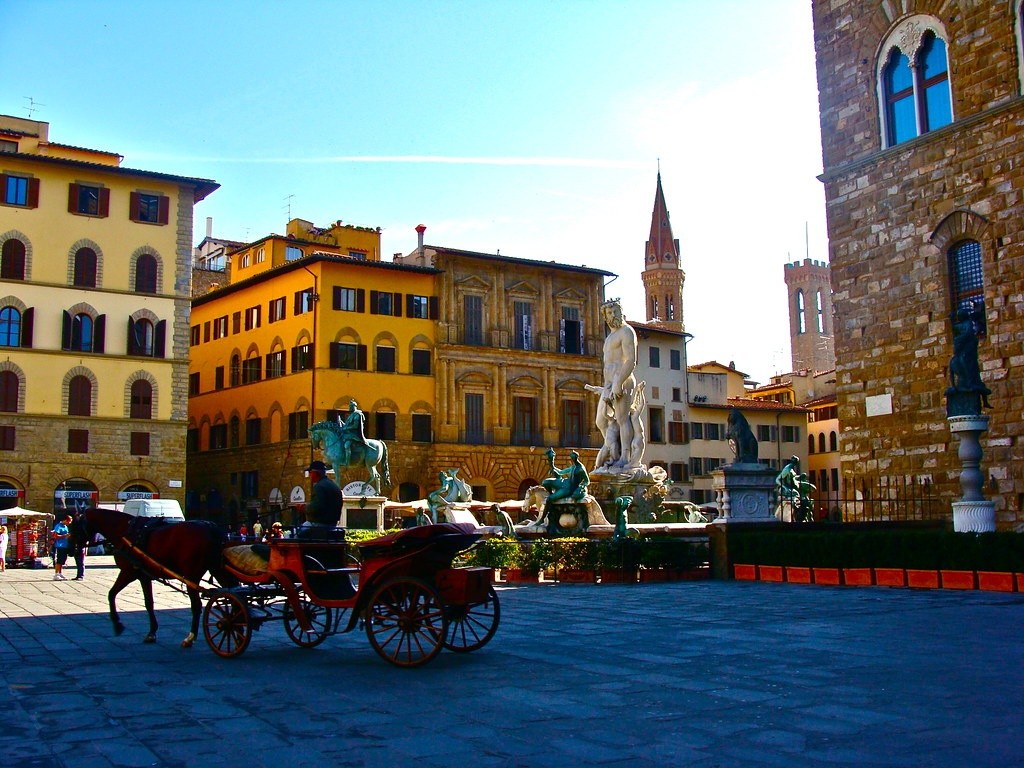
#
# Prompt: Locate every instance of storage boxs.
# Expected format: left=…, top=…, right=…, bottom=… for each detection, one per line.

left=5, top=530, right=42, bottom=569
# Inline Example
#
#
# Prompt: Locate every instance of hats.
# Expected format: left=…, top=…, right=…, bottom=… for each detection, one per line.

left=304, top=461, right=328, bottom=471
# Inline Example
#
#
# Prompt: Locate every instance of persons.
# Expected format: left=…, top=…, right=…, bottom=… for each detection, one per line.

left=0, top=526, right=9, bottom=572
left=286, top=461, right=343, bottom=538
left=417, top=471, right=459, bottom=525
left=272, top=522, right=284, bottom=539
left=776, top=455, right=817, bottom=497
left=595, top=303, right=644, bottom=469
left=240, top=524, right=248, bottom=541
left=52, top=514, right=89, bottom=581
left=228, top=525, right=234, bottom=540
left=949, top=309, right=993, bottom=409
left=94, top=533, right=106, bottom=555
left=253, top=520, right=263, bottom=537
left=534, top=450, right=589, bottom=525
left=340, top=401, right=365, bottom=465
left=490, top=504, right=515, bottom=536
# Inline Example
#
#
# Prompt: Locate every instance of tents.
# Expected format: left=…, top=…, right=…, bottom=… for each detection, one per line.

left=0, top=507, right=51, bottom=516
left=380, top=498, right=536, bottom=516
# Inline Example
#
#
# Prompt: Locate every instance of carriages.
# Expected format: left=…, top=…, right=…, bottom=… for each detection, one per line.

left=69, top=507, right=501, bottom=669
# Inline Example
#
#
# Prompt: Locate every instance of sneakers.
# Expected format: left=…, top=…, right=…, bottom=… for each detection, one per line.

left=53, top=573, right=68, bottom=581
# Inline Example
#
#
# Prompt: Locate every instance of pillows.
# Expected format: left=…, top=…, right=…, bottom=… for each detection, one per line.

left=224, top=544, right=268, bottom=575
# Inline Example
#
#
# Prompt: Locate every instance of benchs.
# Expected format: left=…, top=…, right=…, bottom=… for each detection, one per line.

left=270, top=527, right=347, bottom=547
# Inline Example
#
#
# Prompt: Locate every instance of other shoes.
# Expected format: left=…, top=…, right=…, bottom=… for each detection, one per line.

left=0, top=568, right=6, bottom=573
left=71, top=575, right=84, bottom=581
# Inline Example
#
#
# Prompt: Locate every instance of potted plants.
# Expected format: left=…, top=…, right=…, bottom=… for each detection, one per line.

left=466, top=538, right=712, bottom=582
left=344, top=529, right=361, bottom=574
